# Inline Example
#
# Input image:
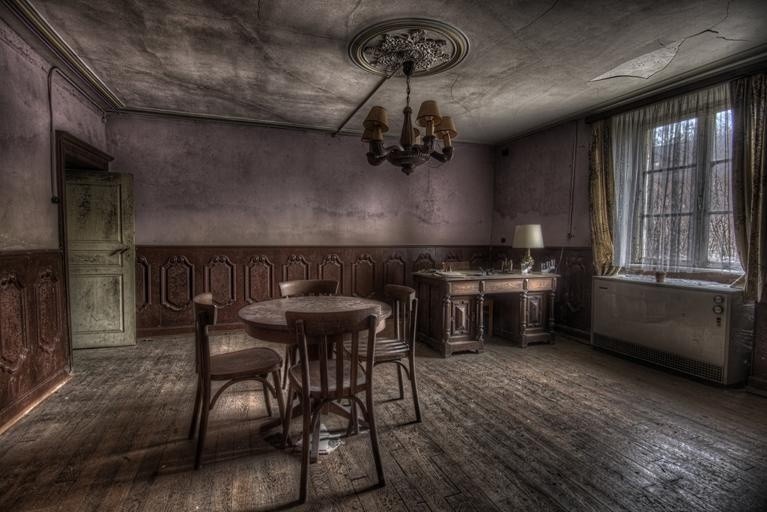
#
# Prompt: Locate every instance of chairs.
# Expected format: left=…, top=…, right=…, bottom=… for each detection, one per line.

left=188, top=288, right=293, bottom=468
left=277, top=277, right=344, bottom=388
left=343, top=282, right=423, bottom=430
left=280, top=302, right=385, bottom=504
left=440, top=260, right=470, bottom=272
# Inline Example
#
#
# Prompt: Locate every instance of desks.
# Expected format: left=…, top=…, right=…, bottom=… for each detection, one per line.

left=237, top=295, right=393, bottom=463
left=410, top=269, right=562, bottom=358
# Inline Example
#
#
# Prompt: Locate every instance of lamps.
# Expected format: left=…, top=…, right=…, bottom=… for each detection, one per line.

left=511, top=223, right=545, bottom=274
left=360, top=61, right=458, bottom=176
